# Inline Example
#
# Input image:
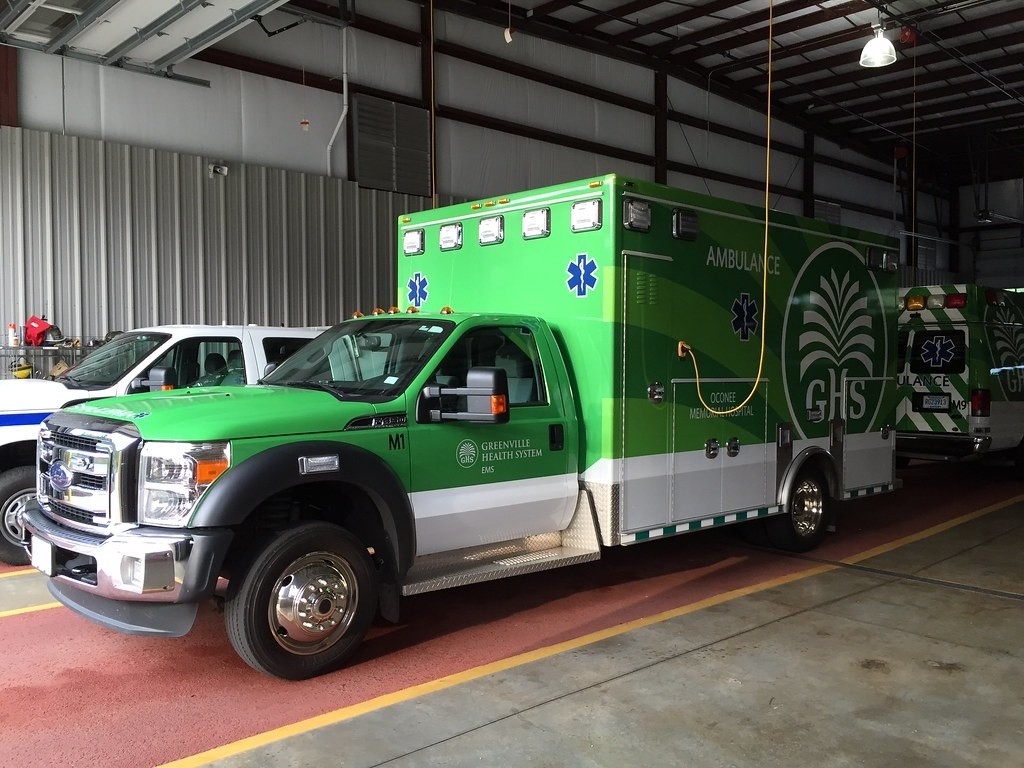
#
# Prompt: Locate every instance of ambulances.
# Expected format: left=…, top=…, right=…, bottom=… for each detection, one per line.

left=21, top=174, right=900, bottom=681
left=896, top=285, right=1024, bottom=471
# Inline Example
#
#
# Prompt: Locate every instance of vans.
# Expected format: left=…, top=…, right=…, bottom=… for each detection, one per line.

left=0, top=324, right=332, bottom=567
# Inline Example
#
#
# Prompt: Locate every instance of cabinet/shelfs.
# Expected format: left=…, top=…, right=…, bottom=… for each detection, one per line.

left=74, top=345, right=97, bottom=365
left=0, top=345, right=73, bottom=381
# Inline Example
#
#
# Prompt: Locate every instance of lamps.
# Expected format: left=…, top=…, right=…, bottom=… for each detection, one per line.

left=859, top=10, right=896, bottom=67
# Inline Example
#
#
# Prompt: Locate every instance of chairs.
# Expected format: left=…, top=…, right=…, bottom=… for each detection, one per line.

left=418, top=347, right=459, bottom=410
left=192, top=353, right=228, bottom=387
left=495, top=354, right=538, bottom=403
left=220, top=350, right=246, bottom=386
